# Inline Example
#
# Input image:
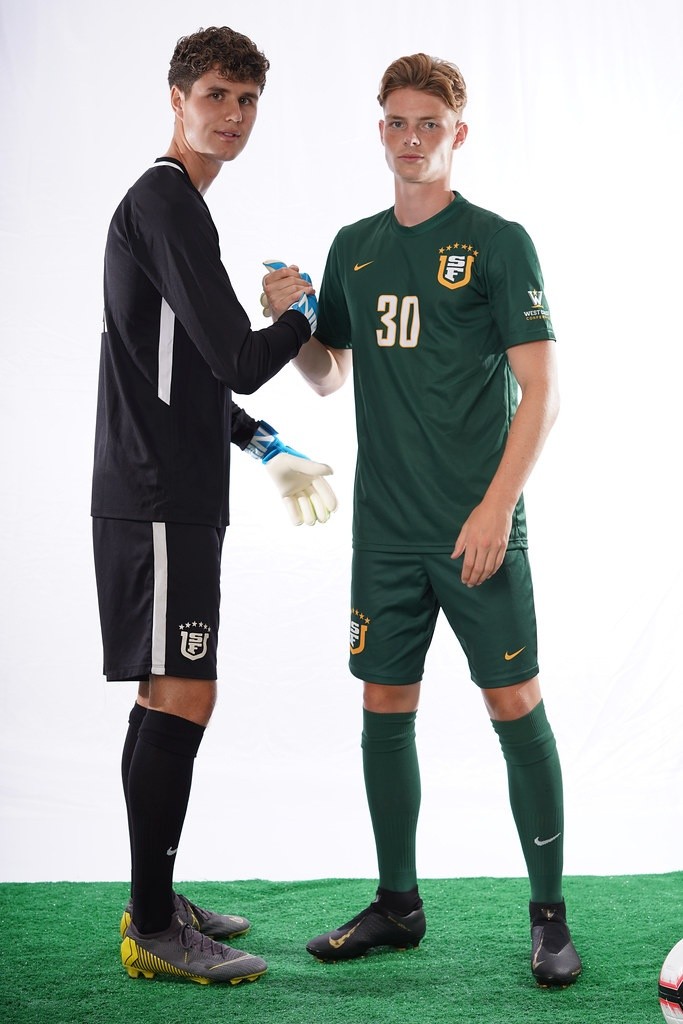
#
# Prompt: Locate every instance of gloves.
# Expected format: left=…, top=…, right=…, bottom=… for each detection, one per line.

left=262, top=258, right=318, bottom=337
left=245, top=420, right=337, bottom=525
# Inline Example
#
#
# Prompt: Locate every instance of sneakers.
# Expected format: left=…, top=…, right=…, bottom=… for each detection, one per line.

left=121, top=917, right=267, bottom=984
left=120, top=892, right=250, bottom=940
left=305, top=896, right=426, bottom=959
left=527, top=906, right=580, bottom=989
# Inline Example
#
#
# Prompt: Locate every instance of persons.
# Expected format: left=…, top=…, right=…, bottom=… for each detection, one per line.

left=259, top=51, right=583, bottom=990
left=89, top=24, right=336, bottom=988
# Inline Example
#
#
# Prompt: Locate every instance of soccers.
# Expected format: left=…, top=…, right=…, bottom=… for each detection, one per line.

left=658, top=938, right=683, bottom=1024
